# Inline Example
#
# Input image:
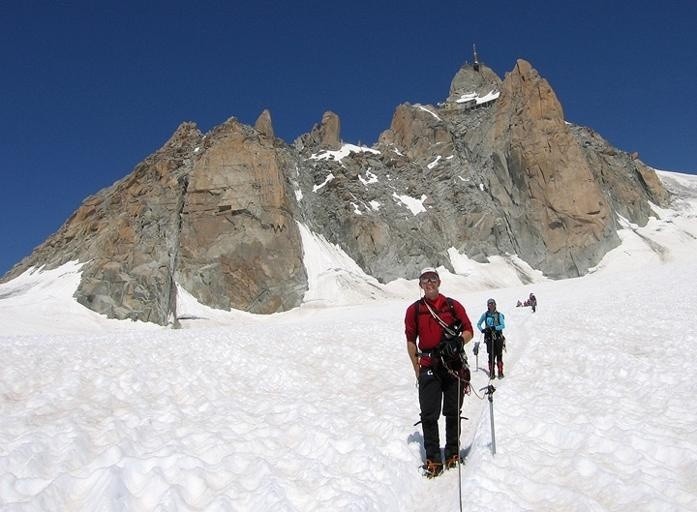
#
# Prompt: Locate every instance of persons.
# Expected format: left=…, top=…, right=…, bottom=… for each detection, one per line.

left=529, top=293, right=536, bottom=312
left=476, top=298, right=506, bottom=380
left=403, top=266, right=473, bottom=477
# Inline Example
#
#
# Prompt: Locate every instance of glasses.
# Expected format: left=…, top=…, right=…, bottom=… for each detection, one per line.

left=420, top=276, right=437, bottom=283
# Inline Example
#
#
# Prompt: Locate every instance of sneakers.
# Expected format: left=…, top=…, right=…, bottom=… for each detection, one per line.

left=426, top=459, right=444, bottom=478
left=446, top=454, right=463, bottom=468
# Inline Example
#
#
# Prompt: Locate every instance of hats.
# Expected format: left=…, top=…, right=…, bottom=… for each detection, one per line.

left=488, top=299, right=495, bottom=304
left=418, top=266, right=439, bottom=279
